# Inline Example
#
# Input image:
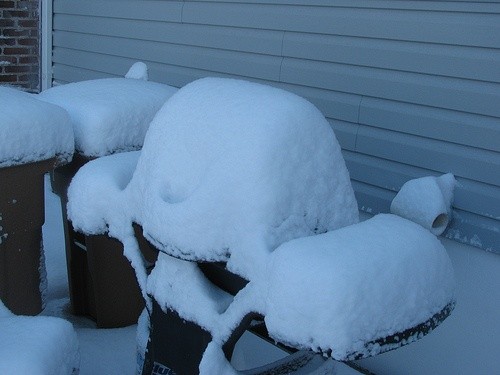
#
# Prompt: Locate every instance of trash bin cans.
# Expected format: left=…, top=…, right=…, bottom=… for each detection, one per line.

left=37, top=76, right=181, bottom=330
left=0, top=84, right=75, bottom=317
